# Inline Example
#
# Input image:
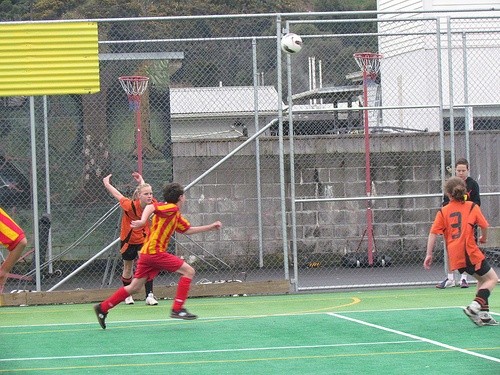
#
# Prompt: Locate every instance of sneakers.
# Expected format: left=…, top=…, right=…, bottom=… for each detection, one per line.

left=436, top=278, right=455, bottom=289
left=94, top=303, right=108, bottom=329
left=460, top=278, right=469, bottom=288
left=146, top=292, right=158, bottom=305
left=463, top=306, right=483, bottom=326
left=479, top=316, right=500, bottom=326
left=171, top=308, right=198, bottom=320
left=125, top=295, right=134, bottom=305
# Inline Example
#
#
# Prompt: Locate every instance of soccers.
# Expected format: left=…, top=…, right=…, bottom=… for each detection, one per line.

left=281, top=33, right=302, bottom=54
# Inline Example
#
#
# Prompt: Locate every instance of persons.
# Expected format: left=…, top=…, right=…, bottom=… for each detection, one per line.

left=103, top=171, right=158, bottom=306
left=423, top=158, right=500, bottom=326
left=0, top=208, right=27, bottom=294
left=95, top=182, right=222, bottom=329
